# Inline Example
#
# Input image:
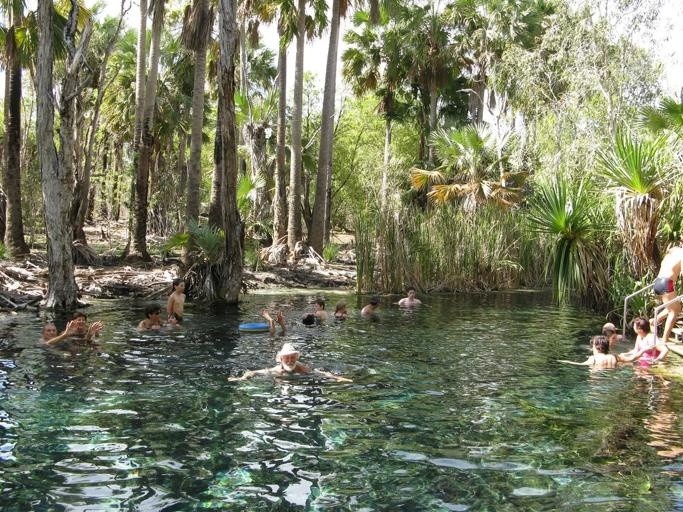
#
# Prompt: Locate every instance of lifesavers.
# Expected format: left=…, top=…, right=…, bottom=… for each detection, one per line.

left=237, top=322, right=269, bottom=333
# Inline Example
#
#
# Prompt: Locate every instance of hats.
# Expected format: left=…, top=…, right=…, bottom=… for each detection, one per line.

left=602, top=321, right=616, bottom=335
left=276, top=344, right=299, bottom=361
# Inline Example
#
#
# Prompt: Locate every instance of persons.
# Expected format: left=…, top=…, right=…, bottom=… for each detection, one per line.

left=360, top=298, right=377, bottom=314
left=137, top=303, right=164, bottom=332
left=620, top=318, right=668, bottom=366
left=38, top=319, right=100, bottom=343
left=60, top=311, right=104, bottom=339
left=604, top=329, right=616, bottom=347
left=259, top=306, right=286, bottom=332
left=229, top=342, right=353, bottom=383
left=649, top=245, right=682, bottom=343
left=166, top=279, right=186, bottom=321
left=333, top=302, right=347, bottom=318
left=561, top=335, right=656, bottom=366
left=602, top=322, right=622, bottom=339
left=303, top=299, right=329, bottom=319
left=398, top=287, right=421, bottom=303
left=164, top=312, right=180, bottom=327
